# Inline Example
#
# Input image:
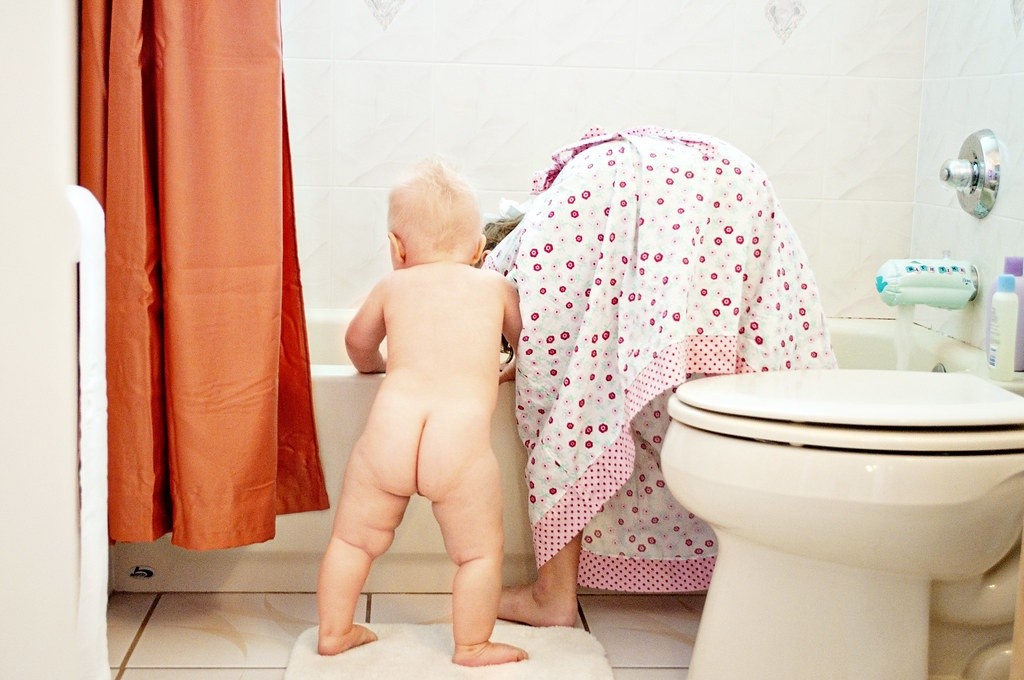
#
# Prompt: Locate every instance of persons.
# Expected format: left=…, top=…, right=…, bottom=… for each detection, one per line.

left=473, top=125, right=839, bottom=629
left=316, top=162, right=530, bottom=666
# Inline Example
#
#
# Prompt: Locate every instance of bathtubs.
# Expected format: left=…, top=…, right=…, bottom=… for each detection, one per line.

left=108, top=303, right=1024, bottom=595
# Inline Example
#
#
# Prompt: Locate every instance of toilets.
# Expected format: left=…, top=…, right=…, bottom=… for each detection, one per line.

left=659, top=366, right=1023, bottom=679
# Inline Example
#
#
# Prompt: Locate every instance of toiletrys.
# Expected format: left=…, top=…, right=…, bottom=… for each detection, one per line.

left=983, top=255, right=1023, bottom=384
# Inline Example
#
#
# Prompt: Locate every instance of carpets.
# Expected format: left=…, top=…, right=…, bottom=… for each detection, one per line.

left=282, top=622, right=616, bottom=680
